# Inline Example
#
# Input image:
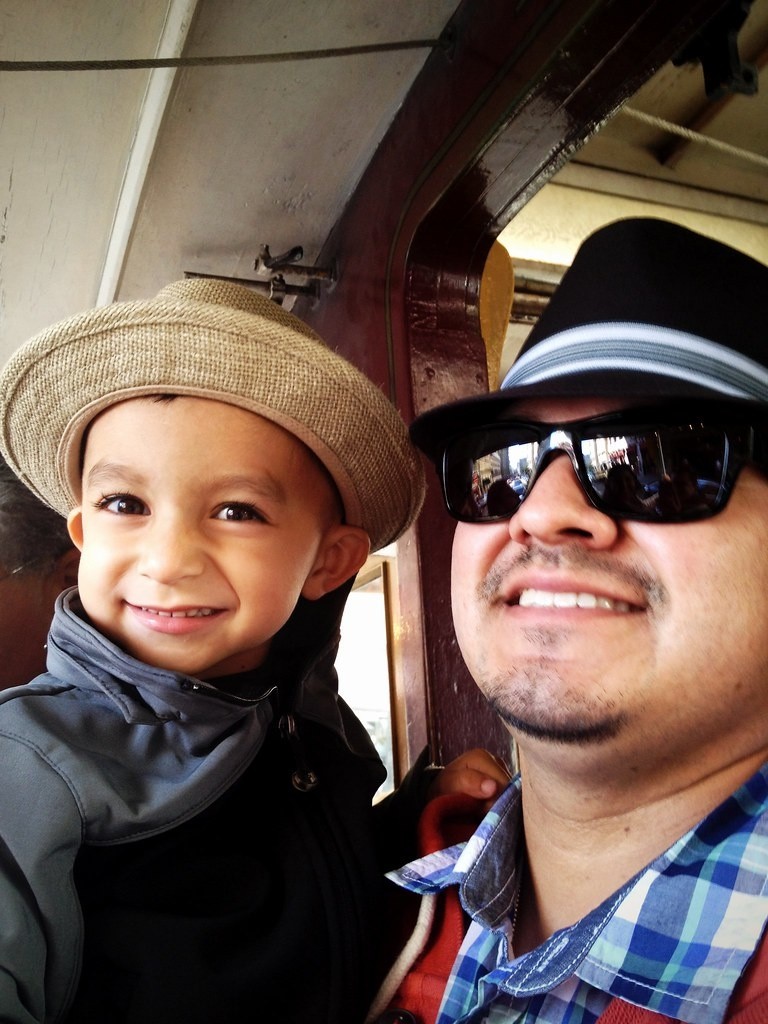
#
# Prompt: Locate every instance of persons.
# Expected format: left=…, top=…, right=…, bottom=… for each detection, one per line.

left=359, top=217, right=768, bottom=1024
left=2, top=277, right=513, bottom=1024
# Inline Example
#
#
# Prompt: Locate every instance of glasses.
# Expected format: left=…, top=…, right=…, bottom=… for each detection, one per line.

left=439, top=403, right=768, bottom=524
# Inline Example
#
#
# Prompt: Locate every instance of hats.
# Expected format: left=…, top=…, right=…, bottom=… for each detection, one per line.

left=0, top=279, right=428, bottom=554
left=404, top=216, right=768, bottom=471
